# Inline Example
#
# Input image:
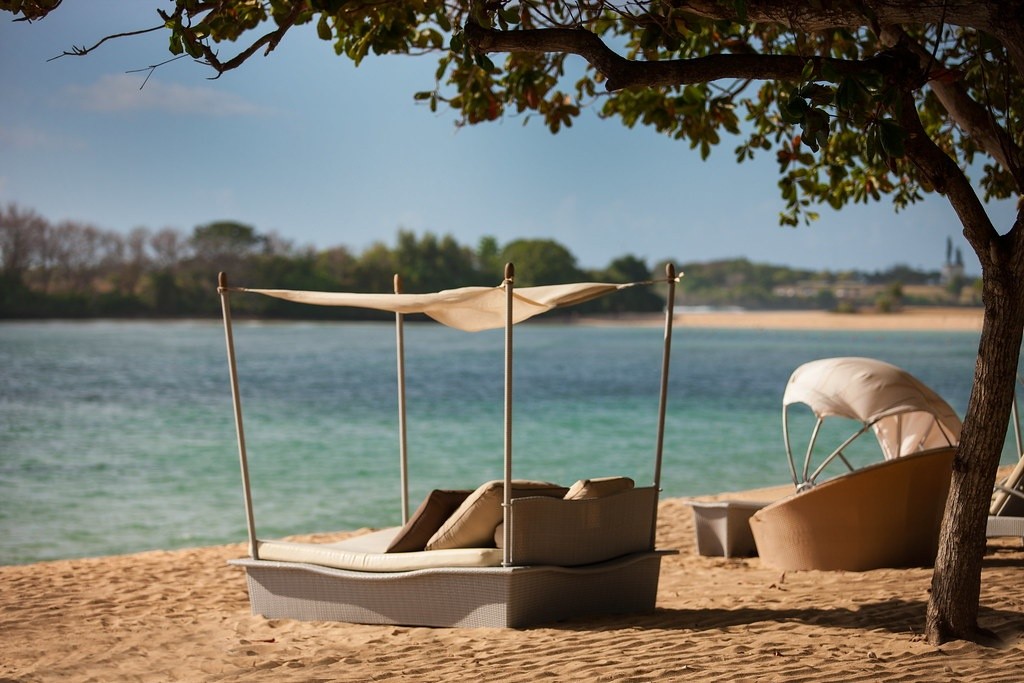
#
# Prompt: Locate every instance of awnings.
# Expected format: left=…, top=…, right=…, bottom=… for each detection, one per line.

left=218, top=263, right=676, bottom=568
left=782, top=357, right=965, bottom=494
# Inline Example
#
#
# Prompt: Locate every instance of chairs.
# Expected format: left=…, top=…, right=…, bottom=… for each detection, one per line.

left=985, top=453, right=1024, bottom=538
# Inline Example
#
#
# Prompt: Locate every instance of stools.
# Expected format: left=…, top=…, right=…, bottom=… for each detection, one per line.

left=679, top=500, right=775, bottom=559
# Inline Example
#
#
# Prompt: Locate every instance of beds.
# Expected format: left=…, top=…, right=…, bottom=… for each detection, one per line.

left=229, top=474, right=680, bottom=629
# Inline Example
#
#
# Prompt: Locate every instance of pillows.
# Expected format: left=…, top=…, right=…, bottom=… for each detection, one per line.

left=561, top=476, right=636, bottom=500
left=424, top=478, right=570, bottom=552
left=382, top=487, right=476, bottom=552
